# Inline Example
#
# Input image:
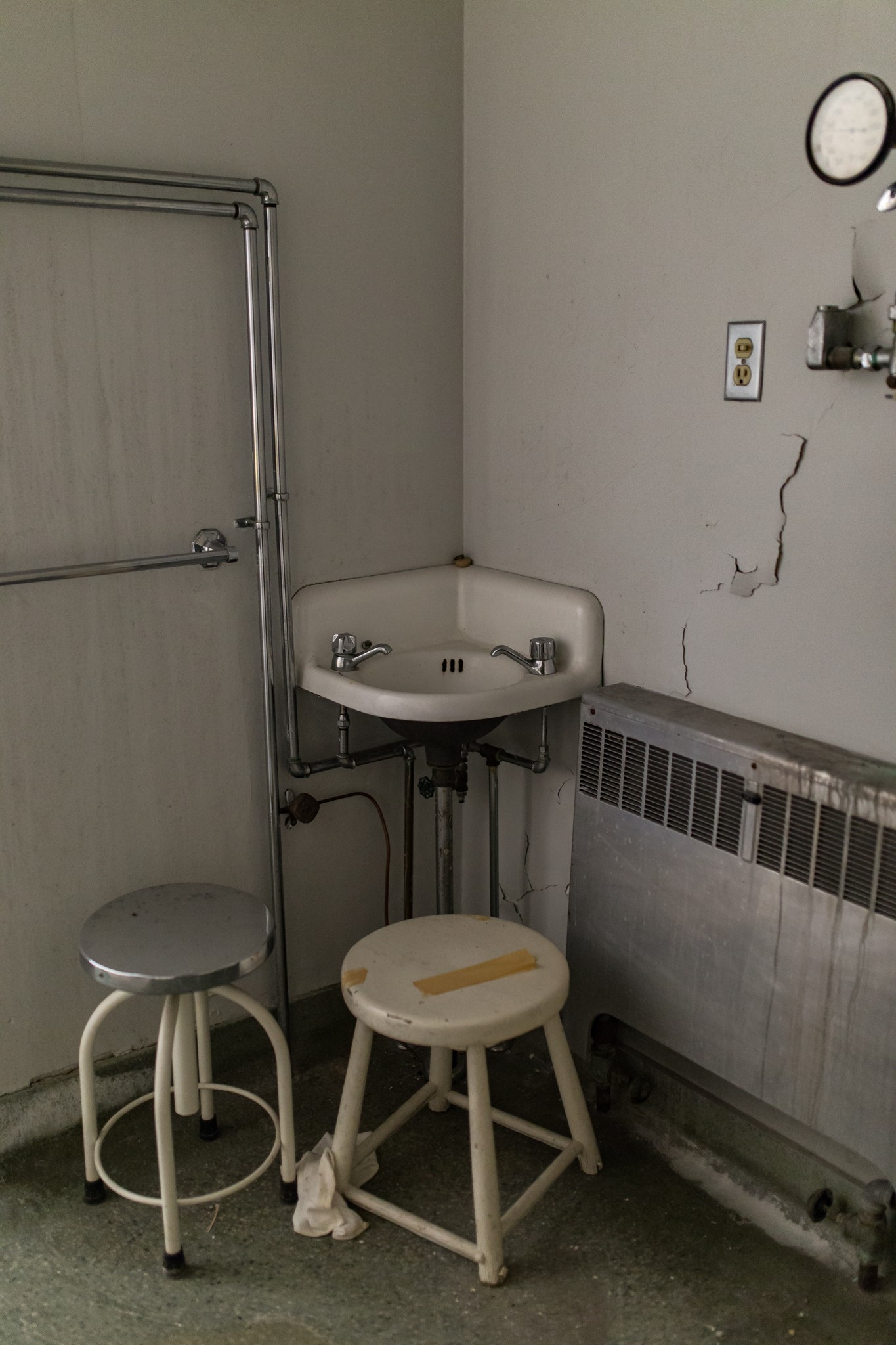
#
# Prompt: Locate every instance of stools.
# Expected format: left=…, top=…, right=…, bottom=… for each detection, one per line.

left=331, top=912, right=600, bottom=1287
left=76, top=882, right=299, bottom=1277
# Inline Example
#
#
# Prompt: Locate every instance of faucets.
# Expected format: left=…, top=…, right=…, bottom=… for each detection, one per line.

left=491, top=636, right=558, bottom=677
left=331, top=632, right=391, bottom=672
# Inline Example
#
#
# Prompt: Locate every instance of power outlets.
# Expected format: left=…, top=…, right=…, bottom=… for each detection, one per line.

left=723, top=321, right=766, bottom=403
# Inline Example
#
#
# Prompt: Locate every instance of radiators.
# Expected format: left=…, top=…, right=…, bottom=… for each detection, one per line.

left=559, top=682, right=896, bottom=1206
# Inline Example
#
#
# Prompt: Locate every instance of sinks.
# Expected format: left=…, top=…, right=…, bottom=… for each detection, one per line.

left=294, top=638, right=583, bottom=746
